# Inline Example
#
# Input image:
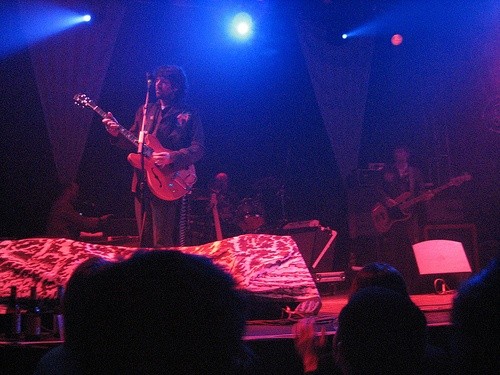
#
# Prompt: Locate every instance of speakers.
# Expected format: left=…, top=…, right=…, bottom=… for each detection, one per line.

left=286, top=227, right=335, bottom=272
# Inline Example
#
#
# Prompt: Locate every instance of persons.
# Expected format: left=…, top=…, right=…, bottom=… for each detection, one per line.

left=33, top=249, right=500, bottom=375
left=376, top=143, right=435, bottom=276
left=102, top=65, right=204, bottom=249
left=47, top=180, right=115, bottom=243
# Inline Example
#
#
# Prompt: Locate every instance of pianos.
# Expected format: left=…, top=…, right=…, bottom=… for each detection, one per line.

left=72, top=214, right=141, bottom=245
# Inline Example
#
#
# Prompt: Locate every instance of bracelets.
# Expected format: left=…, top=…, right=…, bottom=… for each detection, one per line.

left=98, top=216, right=102, bottom=226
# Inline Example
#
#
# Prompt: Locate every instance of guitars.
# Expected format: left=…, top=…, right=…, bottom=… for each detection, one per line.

left=73, top=92, right=198, bottom=201
left=370, top=172, right=472, bottom=234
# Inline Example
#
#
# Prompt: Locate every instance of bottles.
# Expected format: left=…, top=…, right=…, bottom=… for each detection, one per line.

left=24, top=286, right=41, bottom=340
left=5, top=286, right=21, bottom=342
left=54, top=285, right=64, bottom=341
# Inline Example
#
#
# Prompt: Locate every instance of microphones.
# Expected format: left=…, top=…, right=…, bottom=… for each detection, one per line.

left=146, top=72, right=153, bottom=89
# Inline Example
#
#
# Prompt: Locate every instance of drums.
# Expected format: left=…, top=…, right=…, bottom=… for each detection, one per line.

left=235, top=198, right=266, bottom=234
